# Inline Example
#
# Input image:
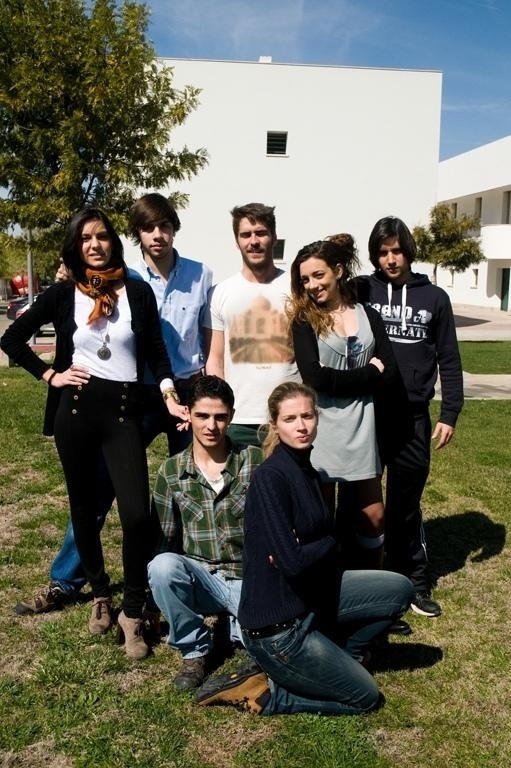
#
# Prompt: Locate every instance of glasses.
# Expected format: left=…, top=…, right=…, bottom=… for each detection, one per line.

left=347, top=336, right=362, bottom=371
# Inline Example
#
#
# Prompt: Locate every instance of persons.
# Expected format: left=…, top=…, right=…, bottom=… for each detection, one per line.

left=340, top=219, right=464, bottom=618
left=142, top=375, right=266, bottom=692
left=192, top=381, right=414, bottom=717
left=0, top=208, right=186, bottom=659
left=10, top=193, right=211, bottom=617
left=200, top=203, right=303, bottom=447
left=286, top=235, right=410, bottom=636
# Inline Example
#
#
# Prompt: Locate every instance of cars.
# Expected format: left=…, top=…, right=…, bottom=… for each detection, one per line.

left=16, top=295, right=57, bottom=336
left=6, top=294, right=37, bottom=320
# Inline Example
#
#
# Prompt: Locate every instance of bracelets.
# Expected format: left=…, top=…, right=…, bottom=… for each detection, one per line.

left=163, top=388, right=180, bottom=402
left=48, top=370, right=57, bottom=384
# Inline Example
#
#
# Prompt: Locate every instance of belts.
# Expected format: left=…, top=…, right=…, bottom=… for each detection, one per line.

left=240, top=618, right=295, bottom=639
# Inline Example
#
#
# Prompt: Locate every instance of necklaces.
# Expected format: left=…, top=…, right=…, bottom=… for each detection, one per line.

left=88, top=294, right=114, bottom=360
left=331, top=303, right=346, bottom=313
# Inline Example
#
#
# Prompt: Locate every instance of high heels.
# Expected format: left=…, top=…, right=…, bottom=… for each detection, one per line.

left=89, top=597, right=111, bottom=633
left=116, top=612, right=149, bottom=660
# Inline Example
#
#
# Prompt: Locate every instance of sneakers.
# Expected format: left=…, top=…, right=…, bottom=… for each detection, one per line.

left=176, top=659, right=270, bottom=716
left=16, top=585, right=79, bottom=614
left=388, top=591, right=442, bottom=635
